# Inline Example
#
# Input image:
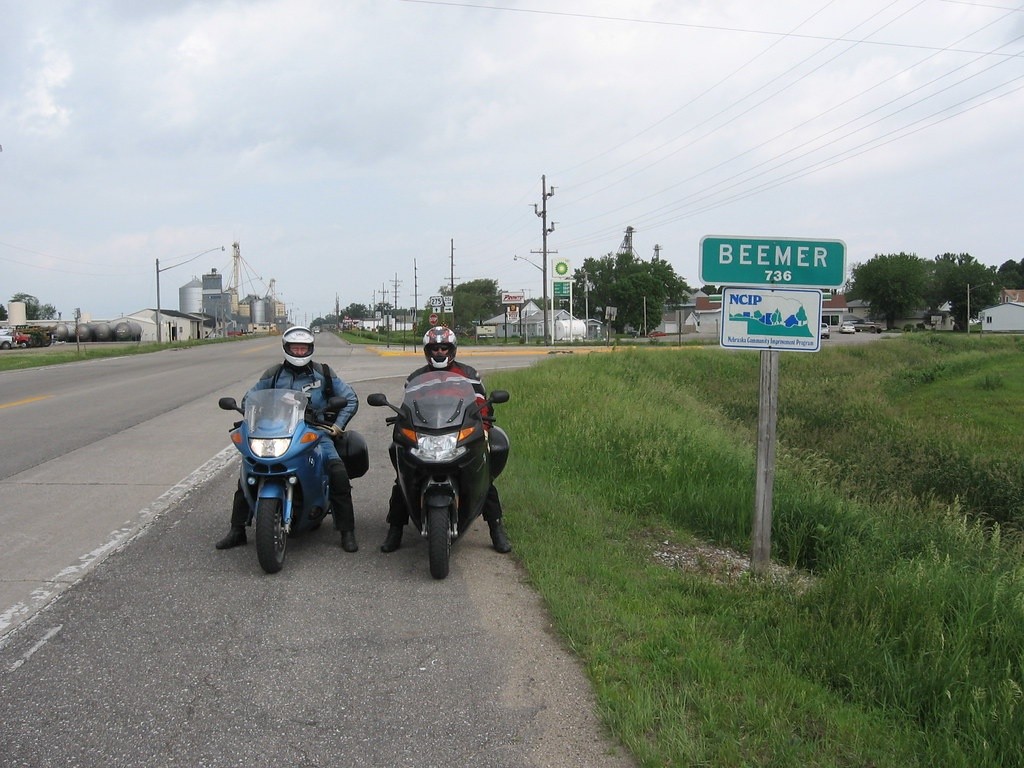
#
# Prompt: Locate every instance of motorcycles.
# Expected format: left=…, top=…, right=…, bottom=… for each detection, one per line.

left=367, top=371, right=510, bottom=579
left=218, top=389, right=369, bottom=573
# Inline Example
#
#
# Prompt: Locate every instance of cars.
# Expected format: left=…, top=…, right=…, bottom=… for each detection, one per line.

left=821, top=323, right=830, bottom=339
left=839, top=321, right=881, bottom=334
left=314, top=328, right=320, bottom=333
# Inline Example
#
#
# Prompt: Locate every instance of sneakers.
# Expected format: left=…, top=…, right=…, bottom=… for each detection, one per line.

left=488, top=519, right=512, bottom=553
left=215, top=528, right=247, bottom=549
left=381, top=524, right=402, bottom=553
left=341, top=531, right=358, bottom=552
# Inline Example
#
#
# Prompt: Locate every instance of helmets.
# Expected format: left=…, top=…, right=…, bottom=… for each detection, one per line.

left=282, top=326, right=315, bottom=366
left=423, top=326, right=458, bottom=368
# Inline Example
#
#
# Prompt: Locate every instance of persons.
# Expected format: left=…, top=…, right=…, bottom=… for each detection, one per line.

left=216, top=326, right=359, bottom=553
left=381, top=326, right=512, bottom=554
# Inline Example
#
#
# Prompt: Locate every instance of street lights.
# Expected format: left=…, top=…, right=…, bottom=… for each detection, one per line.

left=967, top=281, right=994, bottom=333
left=156, top=245, right=225, bottom=343
left=221, top=276, right=262, bottom=337
left=514, top=254, right=549, bottom=346
left=252, top=292, right=283, bottom=335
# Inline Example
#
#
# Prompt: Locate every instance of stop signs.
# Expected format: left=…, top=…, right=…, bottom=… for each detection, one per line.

left=429, top=314, right=438, bottom=325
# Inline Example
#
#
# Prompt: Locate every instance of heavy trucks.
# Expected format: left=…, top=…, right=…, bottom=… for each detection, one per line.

left=0, top=325, right=52, bottom=350
